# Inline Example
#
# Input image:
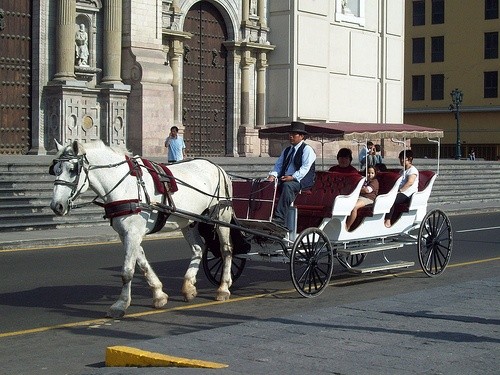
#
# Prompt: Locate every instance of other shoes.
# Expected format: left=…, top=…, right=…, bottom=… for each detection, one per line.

left=269, top=217, right=284, bottom=225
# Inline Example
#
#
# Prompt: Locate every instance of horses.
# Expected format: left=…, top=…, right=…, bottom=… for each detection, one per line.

left=50, top=137, right=234, bottom=319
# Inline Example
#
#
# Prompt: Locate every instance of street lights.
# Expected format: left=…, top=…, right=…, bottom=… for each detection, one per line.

left=447, top=87, right=465, bottom=160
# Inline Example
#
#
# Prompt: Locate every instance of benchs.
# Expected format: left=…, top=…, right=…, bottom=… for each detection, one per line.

left=295, top=171, right=362, bottom=233
left=348, top=171, right=401, bottom=232
left=385, top=169, right=438, bottom=227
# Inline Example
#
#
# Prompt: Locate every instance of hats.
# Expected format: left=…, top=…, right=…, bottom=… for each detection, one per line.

left=284, top=122, right=307, bottom=133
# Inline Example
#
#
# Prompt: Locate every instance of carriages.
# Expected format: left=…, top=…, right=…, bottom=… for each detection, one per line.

left=48, top=121, right=453, bottom=320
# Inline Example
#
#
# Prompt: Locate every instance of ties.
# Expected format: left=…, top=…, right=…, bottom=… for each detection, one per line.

left=283, top=147, right=295, bottom=171
